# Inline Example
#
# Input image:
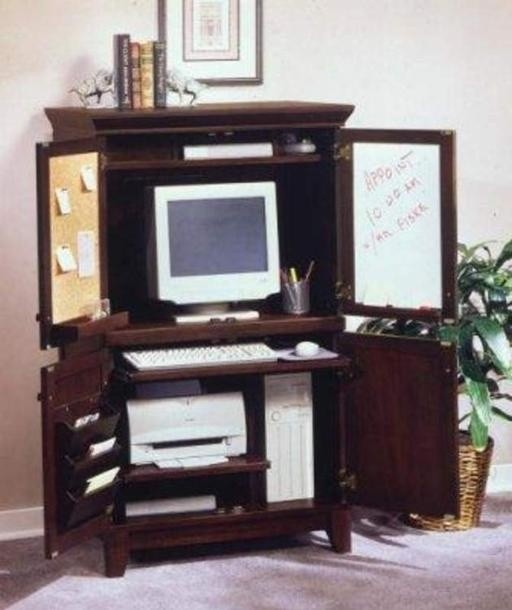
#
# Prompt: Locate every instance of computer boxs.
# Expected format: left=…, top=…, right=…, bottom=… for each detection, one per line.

left=262, top=372, right=315, bottom=502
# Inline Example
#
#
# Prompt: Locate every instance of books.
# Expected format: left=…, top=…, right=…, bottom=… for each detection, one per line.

left=83, top=434, right=117, bottom=460
left=81, top=465, right=122, bottom=499
left=181, top=139, right=275, bottom=159
left=153, top=40, right=170, bottom=108
left=139, top=41, right=155, bottom=109
left=112, top=32, right=132, bottom=111
left=129, top=41, right=141, bottom=111
left=125, top=492, right=218, bottom=521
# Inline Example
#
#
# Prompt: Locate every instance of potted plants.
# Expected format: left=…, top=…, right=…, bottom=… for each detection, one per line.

left=353, top=238, right=512, bottom=533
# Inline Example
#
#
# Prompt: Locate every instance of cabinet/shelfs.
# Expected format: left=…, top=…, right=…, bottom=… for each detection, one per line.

left=36, top=101, right=458, bottom=350
left=41, top=317, right=459, bottom=577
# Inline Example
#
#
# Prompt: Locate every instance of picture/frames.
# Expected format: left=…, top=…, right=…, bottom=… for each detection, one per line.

left=156, top=0, right=263, bottom=87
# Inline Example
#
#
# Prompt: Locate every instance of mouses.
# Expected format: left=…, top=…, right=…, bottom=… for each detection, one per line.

left=296, top=339, right=320, bottom=354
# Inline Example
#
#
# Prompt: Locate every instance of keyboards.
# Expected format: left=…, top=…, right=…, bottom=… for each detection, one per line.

left=123, top=342, right=274, bottom=369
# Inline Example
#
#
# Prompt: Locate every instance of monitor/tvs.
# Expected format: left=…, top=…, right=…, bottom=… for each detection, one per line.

left=145, top=183, right=279, bottom=324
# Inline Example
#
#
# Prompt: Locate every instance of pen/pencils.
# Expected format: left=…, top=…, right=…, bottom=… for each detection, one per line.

left=281, top=259, right=316, bottom=313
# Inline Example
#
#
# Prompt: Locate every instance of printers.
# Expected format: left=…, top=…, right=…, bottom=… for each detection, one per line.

left=124, top=381, right=247, bottom=468
